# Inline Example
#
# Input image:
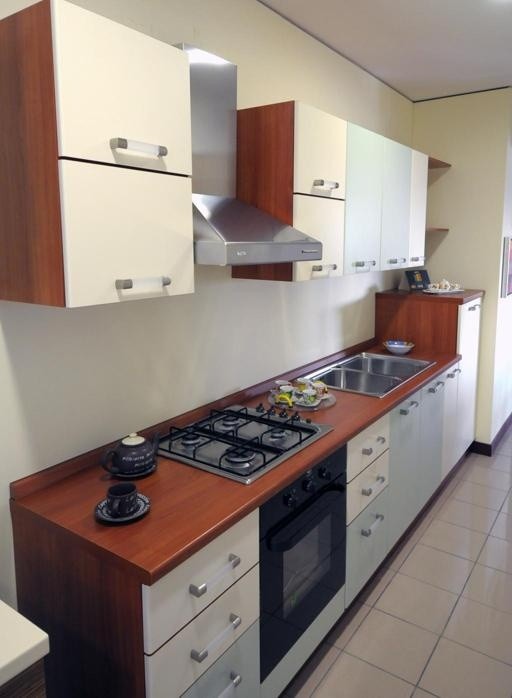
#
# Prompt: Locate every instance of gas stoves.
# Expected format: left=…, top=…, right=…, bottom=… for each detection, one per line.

left=154, top=403, right=336, bottom=485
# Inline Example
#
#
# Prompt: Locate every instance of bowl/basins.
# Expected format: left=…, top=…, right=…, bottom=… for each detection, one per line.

left=382, top=340, right=415, bottom=355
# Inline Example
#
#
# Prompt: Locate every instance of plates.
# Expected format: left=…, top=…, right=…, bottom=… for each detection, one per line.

left=423, top=289, right=464, bottom=294
left=95, top=491, right=150, bottom=525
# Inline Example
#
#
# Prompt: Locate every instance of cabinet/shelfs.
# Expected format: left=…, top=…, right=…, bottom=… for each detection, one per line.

left=375, top=298, right=482, bottom=455
left=12, top=508, right=261, bottom=698
left=1, top=0, right=194, bottom=314
left=347, top=363, right=461, bottom=618
left=236, top=101, right=452, bottom=280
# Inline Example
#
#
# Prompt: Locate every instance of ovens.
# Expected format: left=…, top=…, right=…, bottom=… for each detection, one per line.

left=256, top=441, right=346, bottom=682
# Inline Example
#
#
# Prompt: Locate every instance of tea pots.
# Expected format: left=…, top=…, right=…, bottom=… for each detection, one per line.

left=102, top=430, right=161, bottom=479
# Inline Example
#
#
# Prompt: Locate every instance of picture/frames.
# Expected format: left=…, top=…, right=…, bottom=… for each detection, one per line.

left=501, top=237, right=512, bottom=298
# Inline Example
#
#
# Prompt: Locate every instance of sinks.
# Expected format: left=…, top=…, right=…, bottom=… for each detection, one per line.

left=335, top=351, right=439, bottom=378
left=295, top=366, right=403, bottom=400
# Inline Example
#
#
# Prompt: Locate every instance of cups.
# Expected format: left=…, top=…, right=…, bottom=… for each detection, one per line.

left=428, top=284, right=439, bottom=291
left=275, top=378, right=328, bottom=406
left=106, top=481, right=138, bottom=517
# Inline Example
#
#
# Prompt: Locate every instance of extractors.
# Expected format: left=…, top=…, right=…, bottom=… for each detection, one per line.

left=173, top=41, right=323, bottom=268
